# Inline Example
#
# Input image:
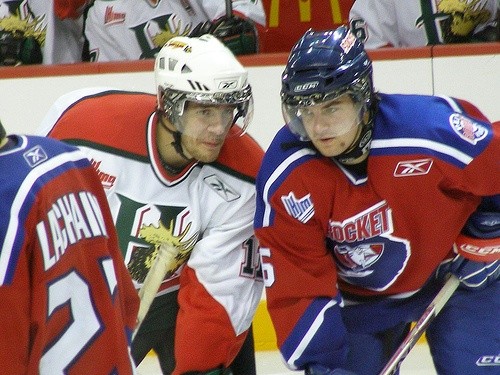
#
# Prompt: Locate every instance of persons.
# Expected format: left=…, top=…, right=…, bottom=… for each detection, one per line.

left=349, top=0, right=500, bottom=49
left=0, top=121, right=141, bottom=375
left=253, top=25, right=500, bottom=375
left=80, top=0, right=266, bottom=63
left=46, top=34, right=265, bottom=375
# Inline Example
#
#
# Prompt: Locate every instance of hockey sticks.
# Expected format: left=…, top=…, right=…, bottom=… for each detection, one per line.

left=379, top=275, right=463, bottom=374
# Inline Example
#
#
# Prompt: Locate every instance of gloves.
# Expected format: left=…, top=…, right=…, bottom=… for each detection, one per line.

left=191, top=15, right=257, bottom=55
left=435, top=206, right=500, bottom=290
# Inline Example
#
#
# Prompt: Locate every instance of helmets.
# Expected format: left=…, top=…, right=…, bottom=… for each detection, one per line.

left=279, top=25, right=373, bottom=139
left=154, top=34, right=253, bottom=143
